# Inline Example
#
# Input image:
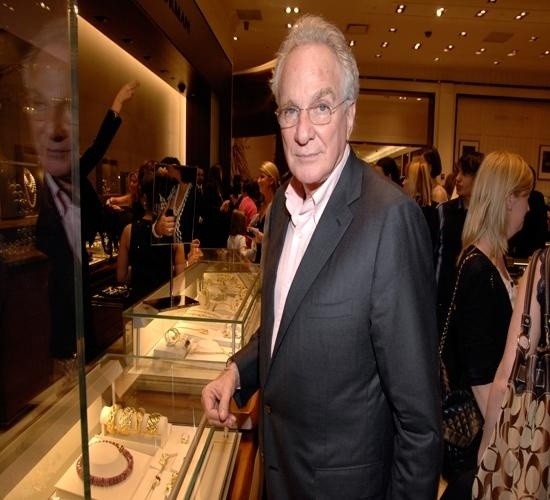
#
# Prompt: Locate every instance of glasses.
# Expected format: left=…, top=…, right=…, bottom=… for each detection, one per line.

left=275, top=99, right=347, bottom=128
left=24, top=96, right=80, bottom=124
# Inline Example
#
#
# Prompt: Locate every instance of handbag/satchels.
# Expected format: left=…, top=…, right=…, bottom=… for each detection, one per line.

left=470, top=346, right=550, bottom=500
left=438, top=355, right=484, bottom=452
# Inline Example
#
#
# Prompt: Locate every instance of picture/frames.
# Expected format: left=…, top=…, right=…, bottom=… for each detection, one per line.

left=458, top=139, right=480, bottom=160
left=536, top=144, right=550, bottom=181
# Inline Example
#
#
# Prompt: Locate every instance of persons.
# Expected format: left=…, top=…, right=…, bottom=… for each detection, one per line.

left=200, top=13, right=444, bottom=500
left=105, top=149, right=549, bottom=500
left=22, top=18, right=81, bottom=359
left=81, top=79, right=139, bottom=364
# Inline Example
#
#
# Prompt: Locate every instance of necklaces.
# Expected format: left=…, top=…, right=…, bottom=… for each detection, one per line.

left=77, top=441, right=134, bottom=486
